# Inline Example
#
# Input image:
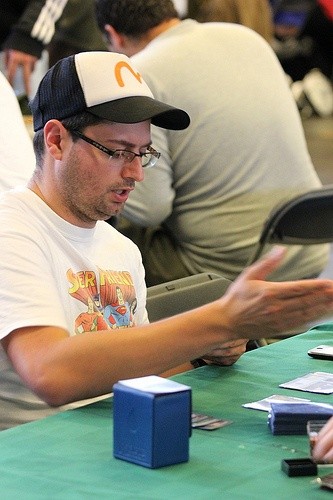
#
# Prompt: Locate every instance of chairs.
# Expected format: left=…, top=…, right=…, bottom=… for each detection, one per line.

left=246, top=185, right=333, bottom=350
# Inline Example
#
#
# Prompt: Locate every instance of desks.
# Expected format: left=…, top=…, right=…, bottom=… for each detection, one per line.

left=0, top=320, right=333, bottom=500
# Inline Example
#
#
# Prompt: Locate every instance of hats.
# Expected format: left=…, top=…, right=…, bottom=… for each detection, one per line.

left=31, top=52, right=190, bottom=132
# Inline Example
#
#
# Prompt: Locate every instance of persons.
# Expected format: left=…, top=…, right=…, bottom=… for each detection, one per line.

left=0, top=1, right=333, bottom=198
left=94, top=0, right=325, bottom=285
left=0, top=52, right=333, bottom=430
left=312, top=415, right=333, bottom=465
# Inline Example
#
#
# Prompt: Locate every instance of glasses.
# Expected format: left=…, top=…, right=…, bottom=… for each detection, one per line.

left=70, top=130, right=160, bottom=168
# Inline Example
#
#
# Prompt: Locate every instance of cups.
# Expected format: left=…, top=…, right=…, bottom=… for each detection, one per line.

left=307, top=420, right=326, bottom=456
